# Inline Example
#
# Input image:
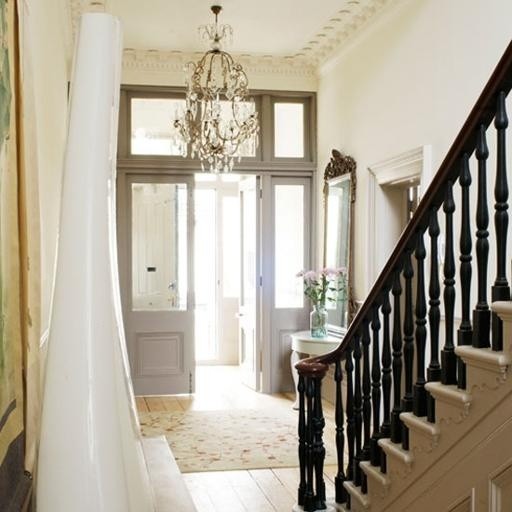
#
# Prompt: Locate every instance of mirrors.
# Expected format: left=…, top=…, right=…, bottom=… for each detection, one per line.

left=313, top=147, right=356, bottom=336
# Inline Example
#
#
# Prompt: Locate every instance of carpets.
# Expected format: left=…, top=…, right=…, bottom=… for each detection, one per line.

left=135, top=413, right=343, bottom=479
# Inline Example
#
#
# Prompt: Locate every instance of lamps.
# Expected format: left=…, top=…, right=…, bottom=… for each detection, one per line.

left=171, top=5, right=260, bottom=175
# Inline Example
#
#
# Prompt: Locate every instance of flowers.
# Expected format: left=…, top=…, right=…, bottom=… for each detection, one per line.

left=296, top=272, right=326, bottom=335
left=325, top=266, right=347, bottom=303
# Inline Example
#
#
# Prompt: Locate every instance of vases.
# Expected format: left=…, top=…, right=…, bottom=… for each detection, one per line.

left=309, top=304, right=329, bottom=337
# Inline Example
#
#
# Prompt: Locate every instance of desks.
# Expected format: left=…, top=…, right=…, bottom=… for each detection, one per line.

left=288, top=329, right=342, bottom=410
